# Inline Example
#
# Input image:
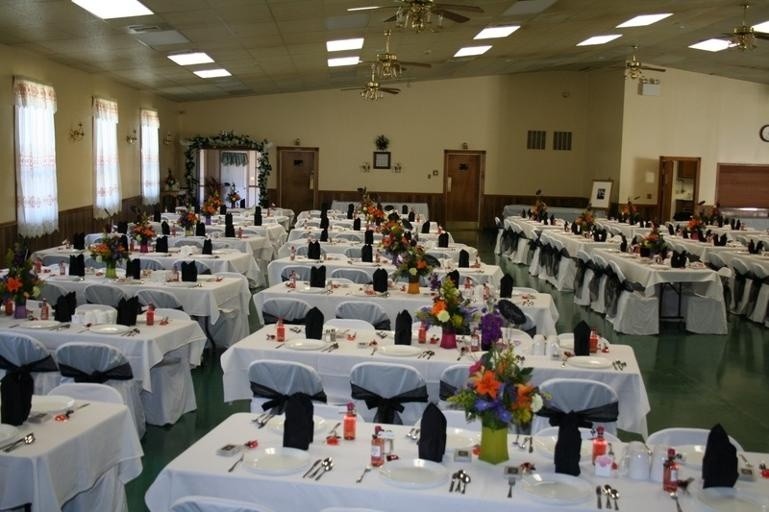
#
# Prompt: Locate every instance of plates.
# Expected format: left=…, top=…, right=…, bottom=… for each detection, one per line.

left=299, top=287, right=327, bottom=293
left=89, top=324, right=129, bottom=334
left=19, top=320, right=61, bottom=329
left=245, top=447, right=309, bottom=476
left=539, top=436, right=595, bottom=458
left=351, top=262, right=378, bottom=266
left=519, top=473, right=594, bottom=504
left=680, top=444, right=705, bottom=468
left=695, top=489, right=767, bottom=511
left=30, top=394, right=76, bottom=413
left=444, top=427, right=478, bottom=449
left=268, top=414, right=329, bottom=436
left=296, top=258, right=318, bottom=262
left=144, top=246, right=233, bottom=258
left=509, top=216, right=670, bottom=270
left=53, top=267, right=218, bottom=287
left=351, top=290, right=383, bottom=297
left=595, top=218, right=769, bottom=260
left=286, top=324, right=614, bottom=369
left=379, top=459, right=450, bottom=489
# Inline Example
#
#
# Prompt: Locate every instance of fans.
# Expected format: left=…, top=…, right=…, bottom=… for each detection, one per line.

left=603, top=45, right=668, bottom=72
left=690, top=0, right=769, bottom=40
left=354, top=28, right=433, bottom=68
left=346, top=0, right=485, bottom=24
left=340, top=62, right=401, bottom=94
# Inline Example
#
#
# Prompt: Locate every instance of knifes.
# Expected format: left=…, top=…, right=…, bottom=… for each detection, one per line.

left=258, top=413, right=279, bottom=428
left=449, top=472, right=457, bottom=493
left=528, top=436, right=535, bottom=454
left=595, top=485, right=602, bottom=509
left=303, top=458, right=322, bottom=478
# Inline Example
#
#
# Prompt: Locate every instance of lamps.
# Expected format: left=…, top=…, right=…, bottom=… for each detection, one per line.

left=624, top=69, right=643, bottom=80
left=395, top=7, right=445, bottom=33
left=361, top=89, right=383, bottom=103
left=378, top=62, right=403, bottom=77
left=728, top=38, right=759, bottom=54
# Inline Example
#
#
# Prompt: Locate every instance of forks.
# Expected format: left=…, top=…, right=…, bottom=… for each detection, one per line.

left=506, top=478, right=517, bottom=498
left=669, top=492, right=682, bottom=511
left=740, top=454, right=753, bottom=468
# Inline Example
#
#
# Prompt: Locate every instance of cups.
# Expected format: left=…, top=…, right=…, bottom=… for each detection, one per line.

left=651, top=447, right=669, bottom=482
left=616, top=441, right=651, bottom=482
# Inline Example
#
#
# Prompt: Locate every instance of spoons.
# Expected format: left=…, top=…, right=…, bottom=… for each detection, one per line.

left=520, top=435, right=527, bottom=450
left=455, top=468, right=473, bottom=494
left=252, top=412, right=271, bottom=424
left=512, top=434, right=519, bottom=446
left=610, top=489, right=622, bottom=510
left=309, top=457, right=333, bottom=478
left=604, top=484, right=613, bottom=509
left=3, top=434, right=36, bottom=453
left=407, top=427, right=422, bottom=440
left=316, top=461, right=335, bottom=480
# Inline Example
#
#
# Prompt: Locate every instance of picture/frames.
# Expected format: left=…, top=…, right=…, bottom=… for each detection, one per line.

left=374, top=151, right=390, bottom=169
left=589, top=178, right=614, bottom=209
left=760, top=124, right=769, bottom=143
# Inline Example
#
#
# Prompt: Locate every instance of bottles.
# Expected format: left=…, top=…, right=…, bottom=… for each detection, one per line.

left=40, top=299, right=48, bottom=319
left=6, top=297, right=14, bottom=316
left=304, top=220, right=307, bottom=230
left=475, top=255, right=480, bottom=268
left=591, top=426, right=609, bottom=468
left=662, top=447, right=680, bottom=492
left=289, top=245, right=295, bottom=260
left=438, top=225, right=442, bottom=234
left=34, top=256, right=41, bottom=274
left=238, top=227, right=242, bottom=238
left=376, top=249, right=380, bottom=262
left=343, top=403, right=359, bottom=440
left=417, top=214, right=420, bottom=222
left=276, top=316, right=285, bottom=341
left=289, top=270, right=297, bottom=288
left=464, top=277, right=471, bottom=289
left=172, top=225, right=176, bottom=234
left=147, top=302, right=153, bottom=326
left=482, top=282, right=489, bottom=300
